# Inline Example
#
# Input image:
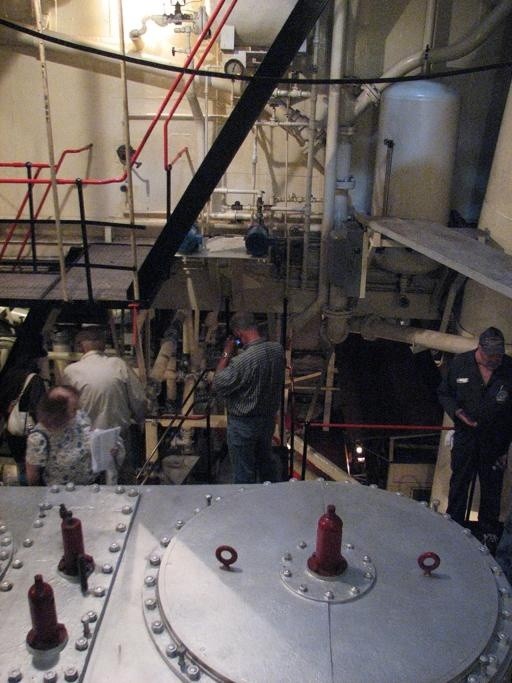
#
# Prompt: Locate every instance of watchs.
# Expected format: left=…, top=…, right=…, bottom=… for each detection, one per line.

left=221, top=352, right=233, bottom=358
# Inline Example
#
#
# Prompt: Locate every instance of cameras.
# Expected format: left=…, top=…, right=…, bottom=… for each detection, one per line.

left=233, top=338, right=243, bottom=346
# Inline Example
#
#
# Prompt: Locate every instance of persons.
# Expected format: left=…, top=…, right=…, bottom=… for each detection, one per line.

left=438, top=326, right=512, bottom=544
left=24, top=385, right=119, bottom=485
left=0, top=333, right=49, bottom=486
left=212, top=311, right=286, bottom=484
left=61, top=330, right=147, bottom=485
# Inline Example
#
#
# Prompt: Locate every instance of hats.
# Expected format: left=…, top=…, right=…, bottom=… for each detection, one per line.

left=478, top=325, right=506, bottom=358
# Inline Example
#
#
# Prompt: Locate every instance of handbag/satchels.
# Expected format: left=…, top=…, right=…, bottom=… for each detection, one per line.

left=8, top=400, right=36, bottom=438
